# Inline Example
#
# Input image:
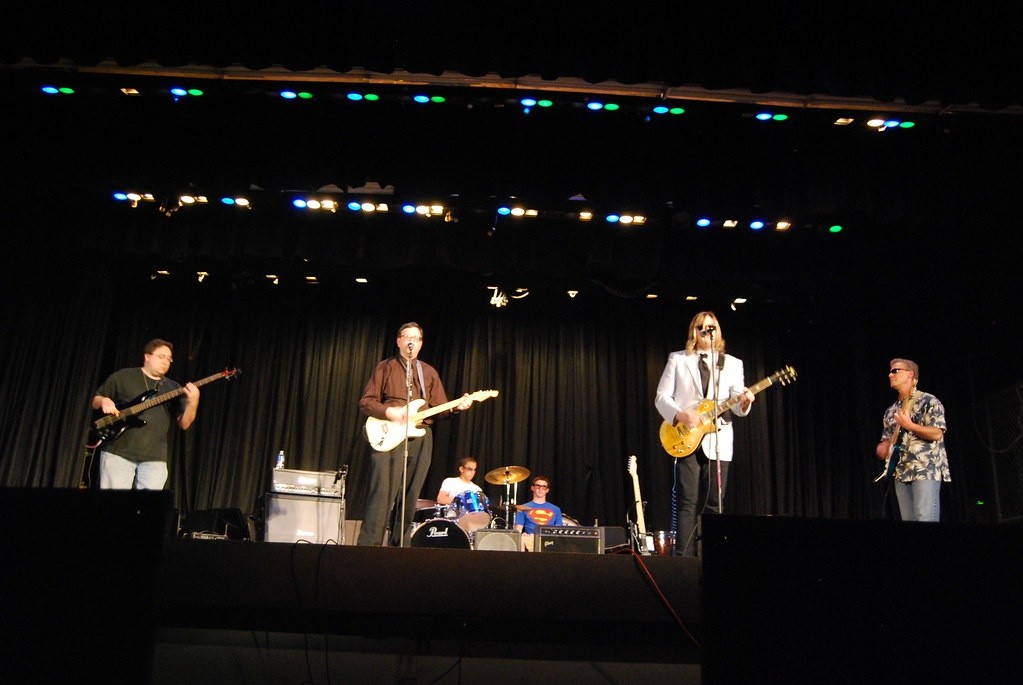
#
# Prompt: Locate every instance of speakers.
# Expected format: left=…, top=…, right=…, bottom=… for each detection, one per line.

left=183, top=507, right=249, bottom=541
left=473, top=528, right=522, bottom=552
left=261, top=493, right=346, bottom=545
left=538, top=526, right=601, bottom=554
left=694, top=514, right=1023, bottom=685
left=0, top=485, right=175, bottom=685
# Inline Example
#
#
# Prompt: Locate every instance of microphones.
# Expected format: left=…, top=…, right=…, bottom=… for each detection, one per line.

left=408, top=343, right=413, bottom=351
left=701, top=329, right=714, bottom=338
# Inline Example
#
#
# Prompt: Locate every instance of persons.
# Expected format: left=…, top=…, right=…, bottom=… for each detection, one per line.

left=515, top=476, right=562, bottom=536
left=437, top=456, right=489, bottom=534
left=358, top=322, right=473, bottom=546
left=655, top=311, right=752, bottom=556
left=90, top=339, right=200, bottom=490
left=876, top=359, right=951, bottom=522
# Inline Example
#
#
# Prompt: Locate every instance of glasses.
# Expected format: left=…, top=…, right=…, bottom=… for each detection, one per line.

left=462, top=465, right=477, bottom=472
left=399, top=334, right=423, bottom=342
left=695, top=324, right=715, bottom=331
left=532, top=484, right=549, bottom=490
left=150, top=351, right=173, bottom=363
left=889, top=368, right=910, bottom=374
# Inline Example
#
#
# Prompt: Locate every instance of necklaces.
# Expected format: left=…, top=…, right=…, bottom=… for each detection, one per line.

left=143, top=371, right=162, bottom=391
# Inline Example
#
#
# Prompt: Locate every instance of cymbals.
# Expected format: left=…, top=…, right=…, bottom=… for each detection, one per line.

left=496, top=504, right=532, bottom=511
left=484, top=466, right=531, bottom=485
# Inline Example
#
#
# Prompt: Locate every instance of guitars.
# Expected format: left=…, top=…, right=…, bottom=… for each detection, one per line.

left=89, top=365, right=244, bottom=443
left=659, top=365, right=798, bottom=459
left=365, top=390, right=499, bottom=453
left=627, top=455, right=656, bottom=556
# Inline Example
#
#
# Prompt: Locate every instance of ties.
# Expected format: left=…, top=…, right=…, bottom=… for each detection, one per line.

left=698, top=353, right=711, bottom=399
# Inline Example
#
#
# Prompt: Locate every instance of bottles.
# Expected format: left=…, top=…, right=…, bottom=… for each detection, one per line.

left=275, top=450, right=285, bottom=469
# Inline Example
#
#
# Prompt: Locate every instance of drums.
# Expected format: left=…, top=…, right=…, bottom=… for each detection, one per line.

left=451, top=489, right=492, bottom=534
left=561, top=513, right=580, bottom=527
left=648, top=530, right=695, bottom=557
left=411, top=503, right=459, bottom=526
left=410, top=518, right=474, bottom=550
